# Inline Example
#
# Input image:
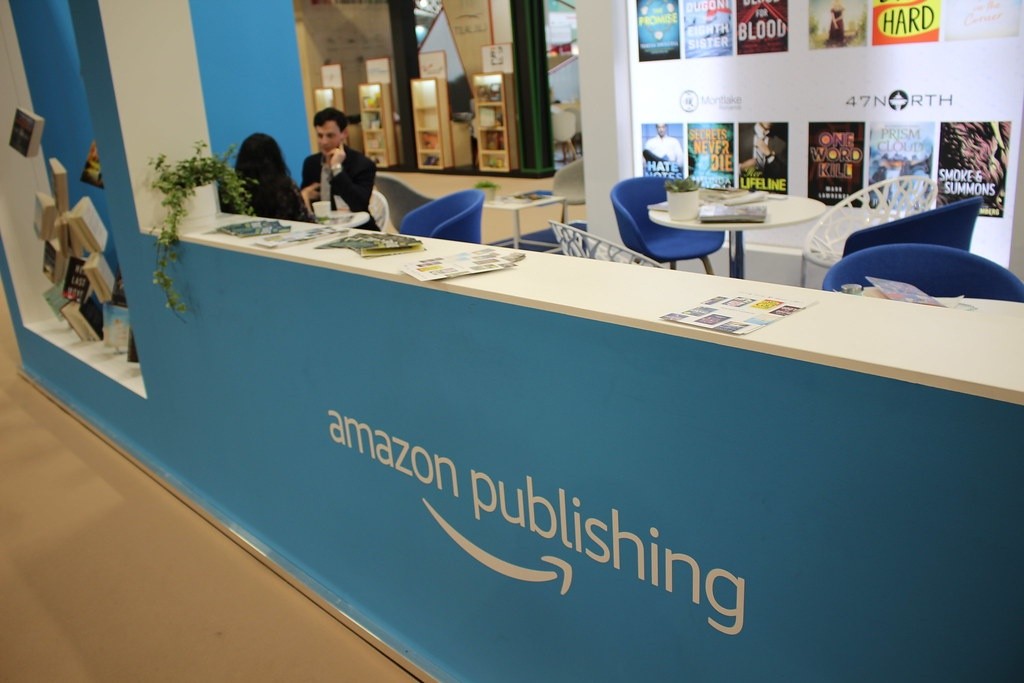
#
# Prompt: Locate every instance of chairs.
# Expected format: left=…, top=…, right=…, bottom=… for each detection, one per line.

left=609, top=177, right=725, bottom=276
left=843, top=197, right=983, bottom=258
left=800, top=175, right=938, bottom=289
left=822, top=243, right=1024, bottom=302
left=550, top=221, right=662, bottom=268
left=400, top=188, right=484, bottom=243
left=553, top=157, right=585, bottom=223
left=374, top=175, right=435, bottom=234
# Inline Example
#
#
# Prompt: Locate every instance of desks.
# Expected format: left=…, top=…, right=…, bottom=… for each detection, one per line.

left=649, top=188, right=826, bottom=279
left=481, top=194, right=567, bottom=254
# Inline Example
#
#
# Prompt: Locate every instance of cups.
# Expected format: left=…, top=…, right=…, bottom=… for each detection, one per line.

left=312, top=200, right=332, bottom=219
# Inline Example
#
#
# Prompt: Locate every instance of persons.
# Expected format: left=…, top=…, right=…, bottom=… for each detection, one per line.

left=217, top=133, right=321, bottom=223
left=646, top=123, right=684, bottom=170
left=739, top=122, right=788, bottom=179
left=827, top=0, right=846, bottom=41
left=300, top=107, right=381, bottom=232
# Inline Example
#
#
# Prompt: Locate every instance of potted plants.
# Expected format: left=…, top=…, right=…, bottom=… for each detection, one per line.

left=148, top=141, right=259, bottom=323
left=665, top=177, right=702, bottom=222
left=475, top=180, right=501, bottom=200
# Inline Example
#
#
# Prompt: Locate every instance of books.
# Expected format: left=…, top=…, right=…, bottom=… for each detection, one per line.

left=863, top=276, right=948, bottom=308
left=9, top=108, right=45, bottom=157
left=647, top=189, right=769, bottom=223
left=79, top=141, right=104, bottom=189
left=33, top=158, right=139, bottom=363
